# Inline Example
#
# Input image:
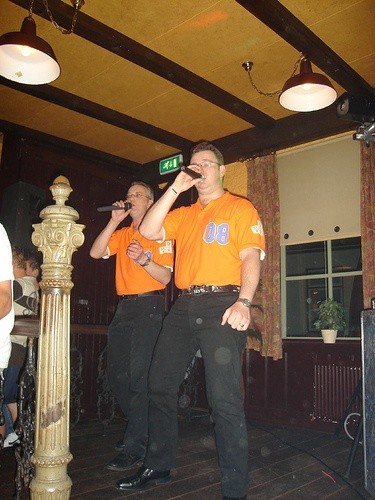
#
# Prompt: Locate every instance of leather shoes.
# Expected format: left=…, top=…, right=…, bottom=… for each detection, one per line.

left=117, top=464, right=171, bottom=491
left=106, top=452, right=144, bottom=471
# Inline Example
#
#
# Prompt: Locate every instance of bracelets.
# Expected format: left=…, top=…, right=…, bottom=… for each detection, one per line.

left=169, top=186, right=180, bottom=196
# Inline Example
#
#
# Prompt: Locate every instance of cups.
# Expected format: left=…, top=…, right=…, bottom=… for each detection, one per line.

left=371, top=298, right=375, bottom=309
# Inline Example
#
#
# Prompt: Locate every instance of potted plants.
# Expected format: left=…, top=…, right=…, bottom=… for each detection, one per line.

left=314, top=298, right=346, bottom=344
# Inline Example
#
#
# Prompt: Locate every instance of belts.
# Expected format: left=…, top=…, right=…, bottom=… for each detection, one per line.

left=178, top=284, right=240, bottom=297
left=120, top=290, right=163, bottom=300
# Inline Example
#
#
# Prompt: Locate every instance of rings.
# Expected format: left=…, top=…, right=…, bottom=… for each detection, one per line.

left=239, top=323, right=244, bottom=327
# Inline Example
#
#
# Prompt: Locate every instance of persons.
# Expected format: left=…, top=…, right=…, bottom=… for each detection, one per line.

left=0, top=222, right=45, bottom=448
left=90, top=182, right=175, bottom=471
left=115, top=143, right=266, bottom=500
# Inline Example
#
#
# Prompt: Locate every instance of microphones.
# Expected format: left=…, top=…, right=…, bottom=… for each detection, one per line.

left=97, top=202, right=132, bottom=212
left=181, top=166, right=204, bottom=181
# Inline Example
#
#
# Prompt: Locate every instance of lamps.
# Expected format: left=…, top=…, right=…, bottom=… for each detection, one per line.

left=278, top=60, right=338, bottom=114
left=0, top=0, right=61, bottom=85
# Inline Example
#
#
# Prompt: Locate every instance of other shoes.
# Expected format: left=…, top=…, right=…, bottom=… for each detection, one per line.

left=2, top=432, right=20, bottom=447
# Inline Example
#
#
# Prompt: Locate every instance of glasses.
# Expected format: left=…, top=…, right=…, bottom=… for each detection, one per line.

left=199, top=160, right=221, bottom=168
left=125, top=193, right=149, bottom=199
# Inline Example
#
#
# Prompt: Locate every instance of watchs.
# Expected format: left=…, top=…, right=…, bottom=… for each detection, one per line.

left=139, top=256, right=151, bottom=267
left=237, top=298, right=252, bottom=307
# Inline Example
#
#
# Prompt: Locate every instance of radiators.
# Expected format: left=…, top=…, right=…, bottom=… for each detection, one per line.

left=313, top=363, right=362, bottom=427
